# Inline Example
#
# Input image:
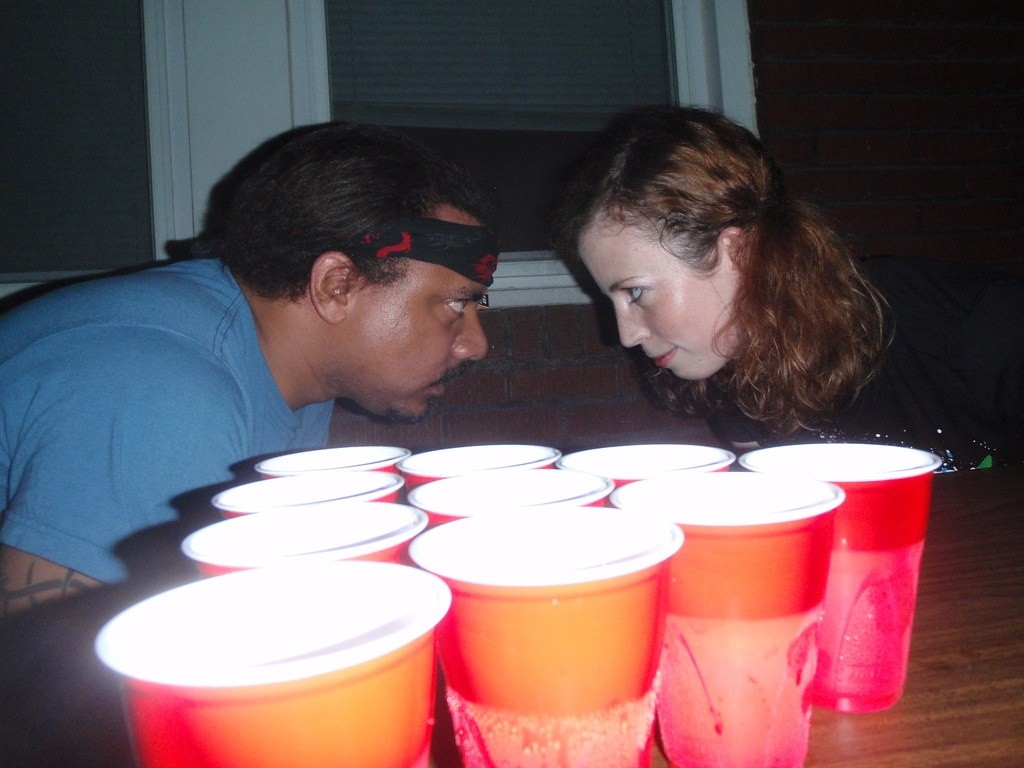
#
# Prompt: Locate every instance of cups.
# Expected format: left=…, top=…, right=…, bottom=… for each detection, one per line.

left=254, top=447, right=411, bottom=476
left=737, top=442, right=942, bottom=714
left=555, top=444, right=736, bottom=489
left=95, top=559, right=452, bottom=768
left=608, top=471, right=846, bottom=768
left=408, top=506, right=686, bottom=768
left=406, top=469, right=616, bottom=531
left=181, top=502, right=429, bottom=580
left=212, top=470, right=405, bottom=519
left=395, top=445, right=561, bottom=492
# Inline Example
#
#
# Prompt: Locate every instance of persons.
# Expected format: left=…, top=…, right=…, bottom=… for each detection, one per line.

left=548, top=101, right=1022, bottom=471
left=0, top=123, right=504, bottom=626
